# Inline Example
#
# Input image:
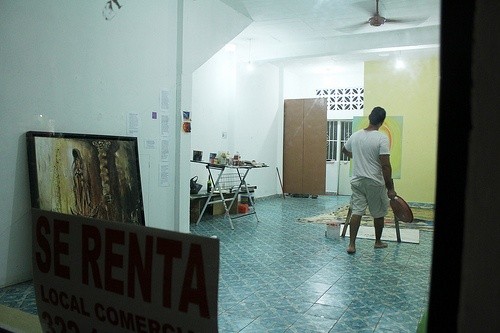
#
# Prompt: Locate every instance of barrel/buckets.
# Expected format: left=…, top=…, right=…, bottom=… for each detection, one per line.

left=325, top=222, right=341, bottom=241
left=193, top=150, right=204, bottom=161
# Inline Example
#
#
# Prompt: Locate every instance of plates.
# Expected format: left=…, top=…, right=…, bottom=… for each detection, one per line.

left=389, top=195, right=414, bottom=223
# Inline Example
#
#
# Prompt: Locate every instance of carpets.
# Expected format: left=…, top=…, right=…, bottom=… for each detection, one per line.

left=298, top=202, right=434, bottom=232
left=340, top=223, right=419, bottom=244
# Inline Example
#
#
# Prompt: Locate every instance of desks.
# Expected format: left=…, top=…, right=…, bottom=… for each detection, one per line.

left=190, top=160, right=270, bottom=230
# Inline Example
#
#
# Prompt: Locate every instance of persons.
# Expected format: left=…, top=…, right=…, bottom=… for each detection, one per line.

left=342, top=106, right=397, bottom=254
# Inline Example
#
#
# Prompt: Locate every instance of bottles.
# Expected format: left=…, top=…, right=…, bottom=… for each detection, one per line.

left=207, top=175, right=212, bottom=193
left=234, top=152, right=240, bottom=160
left=220, top=152, right=227, bottom=164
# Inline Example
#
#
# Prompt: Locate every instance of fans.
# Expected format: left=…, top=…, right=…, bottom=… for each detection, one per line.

left=335, top=0, right=427, bottom=33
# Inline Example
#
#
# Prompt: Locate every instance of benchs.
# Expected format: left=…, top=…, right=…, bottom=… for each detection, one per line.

left=190, top=189, right=254, bottom=224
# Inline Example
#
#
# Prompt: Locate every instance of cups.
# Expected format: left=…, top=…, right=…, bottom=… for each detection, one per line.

left=214, top=157, right=219, bottom=164
left=233, top=160, right=239, bottom=165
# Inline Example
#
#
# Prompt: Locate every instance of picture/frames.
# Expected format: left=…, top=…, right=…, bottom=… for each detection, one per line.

left=26, top=130, right=146, bottom=227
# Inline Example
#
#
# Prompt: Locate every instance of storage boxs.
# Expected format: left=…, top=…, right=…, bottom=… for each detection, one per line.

left=241, top=196, right=255, bottom=206
